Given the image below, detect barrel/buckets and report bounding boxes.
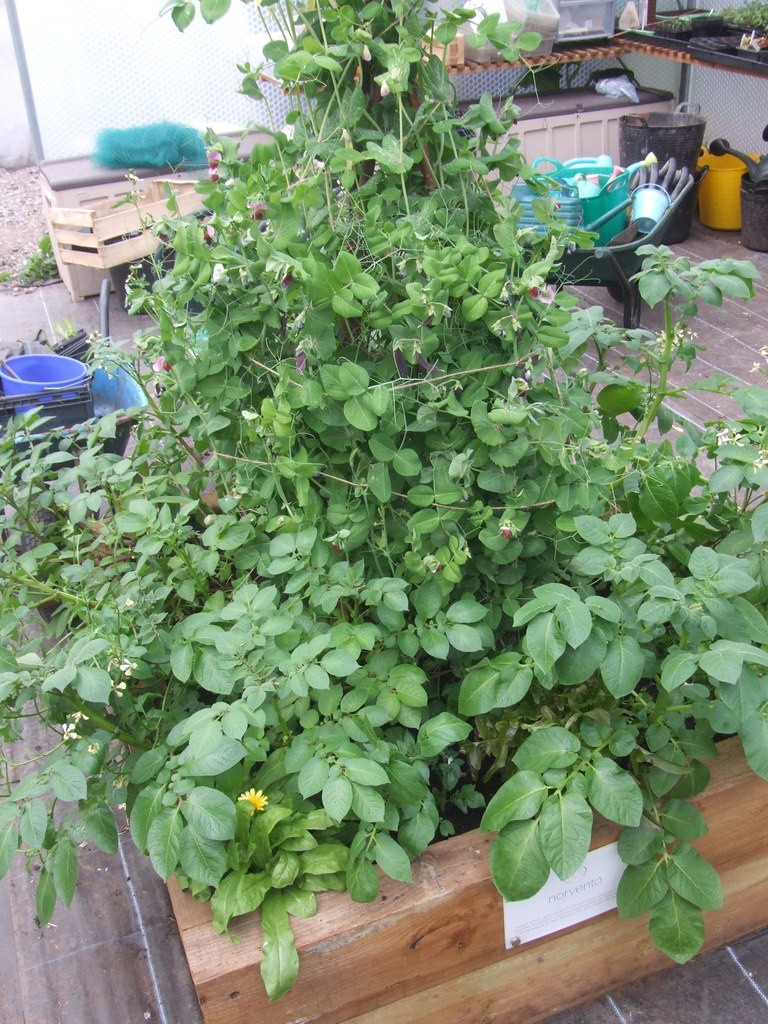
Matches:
[0,354,91,417]
[740,170,768,253]
[696,145,762,231]
[631,165,710,245]
[630,184,671,234]
[531,157,632,247]
[617,101,707,182]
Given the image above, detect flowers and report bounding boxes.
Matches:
[1,0,768,1002]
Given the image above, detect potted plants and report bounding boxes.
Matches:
[651,19,693,42]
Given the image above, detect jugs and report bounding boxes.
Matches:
[710,138,768,182]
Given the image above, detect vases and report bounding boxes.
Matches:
[162,728,766,1024]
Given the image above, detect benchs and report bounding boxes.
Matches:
[37,128,279,303]
[454,82,675,201]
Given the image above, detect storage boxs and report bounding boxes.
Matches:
[47,177,209,268]
[420,0,561,67]
